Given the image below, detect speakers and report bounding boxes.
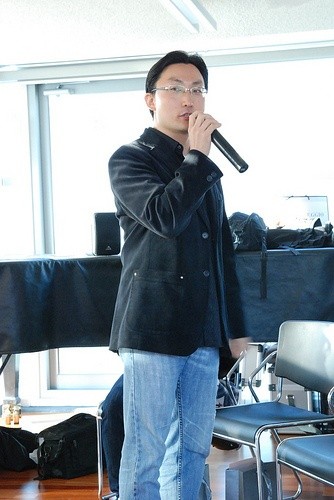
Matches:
[93,212,121,256]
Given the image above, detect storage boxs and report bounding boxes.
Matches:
[225,460,278,500]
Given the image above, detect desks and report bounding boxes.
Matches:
[0,231,334,373]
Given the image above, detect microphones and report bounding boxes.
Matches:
[211,130,248,172]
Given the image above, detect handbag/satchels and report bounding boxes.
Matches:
[0,425,40,471]
[29,412,106,481]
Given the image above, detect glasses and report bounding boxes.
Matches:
[151,84,209,100]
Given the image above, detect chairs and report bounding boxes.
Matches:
[96,320,334,500]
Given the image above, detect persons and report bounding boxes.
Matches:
[108,51,251,500]
